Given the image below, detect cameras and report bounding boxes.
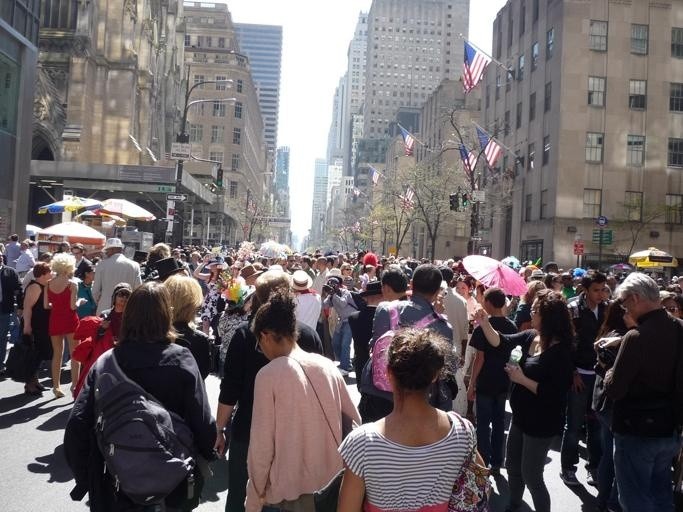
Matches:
[324,285,335,292]
[592,341,618,380]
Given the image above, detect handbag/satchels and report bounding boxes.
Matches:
[6,334,39,382]
[94,374,195,505]
[448,457,492,512]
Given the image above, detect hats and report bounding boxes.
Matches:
[208,257,224,266]
[222,276,256,311]
[289,270,313,291]
[527,269,545,282]
[359,281,382,297]
[101,238,125,253]
[241,265,264,279]
[156,256,184,280]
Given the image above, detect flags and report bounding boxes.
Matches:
[350,187,362,197]
[399,128,415,157]
[462,44,491,93]
[400,187,414,209]
[368,167,381,185]
[460,145,478,175]
[484,140,501,167]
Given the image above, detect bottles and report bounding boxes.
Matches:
[508,345,522,364]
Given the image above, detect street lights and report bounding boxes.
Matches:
[170,79,237,250]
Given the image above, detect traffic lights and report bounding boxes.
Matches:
[216,169,222,187]
[461,192,467,206]
[448,194,458,211]
[470,214,476,227]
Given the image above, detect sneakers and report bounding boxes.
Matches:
[560,471,579,486]
[587,468,600,485]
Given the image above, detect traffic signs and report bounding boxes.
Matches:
[165,193,188,202]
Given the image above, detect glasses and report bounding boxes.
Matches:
[254,332,268,354]
[618,294,639,311]
[666,306,680,313]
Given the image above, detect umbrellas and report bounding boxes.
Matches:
[38,222,106,244]
[103,198,155,222]
[38,196,102,222]
[76,210,129,228]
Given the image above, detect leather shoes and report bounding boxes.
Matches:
[25,385,51,394]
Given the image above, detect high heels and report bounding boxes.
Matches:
[53,388,64,399]
[70,384,77,397]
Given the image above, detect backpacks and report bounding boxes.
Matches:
[373,306,438,393]
